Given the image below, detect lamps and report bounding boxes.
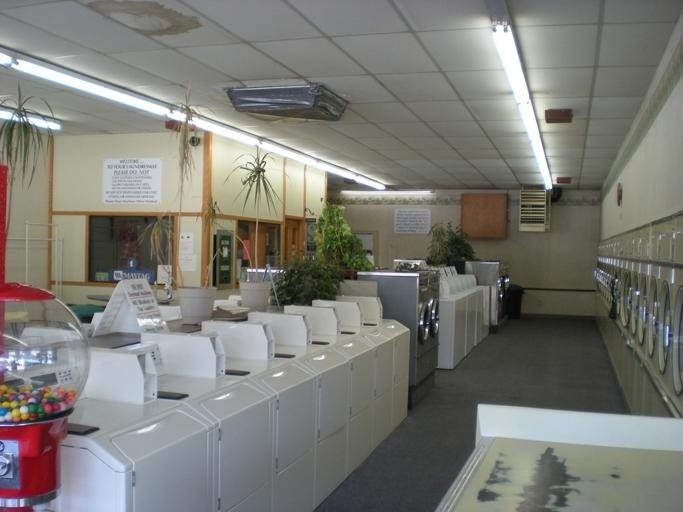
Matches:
[353,176,386,191]
[0,108,62,132]
[530,140,553,191]
[491,23,530,103]
[9,53,171,119]
[0,50,15,68]
[315,161,356,182]
[515,101,541,141]
[169,107,260,147]
[256,138,317,167]
